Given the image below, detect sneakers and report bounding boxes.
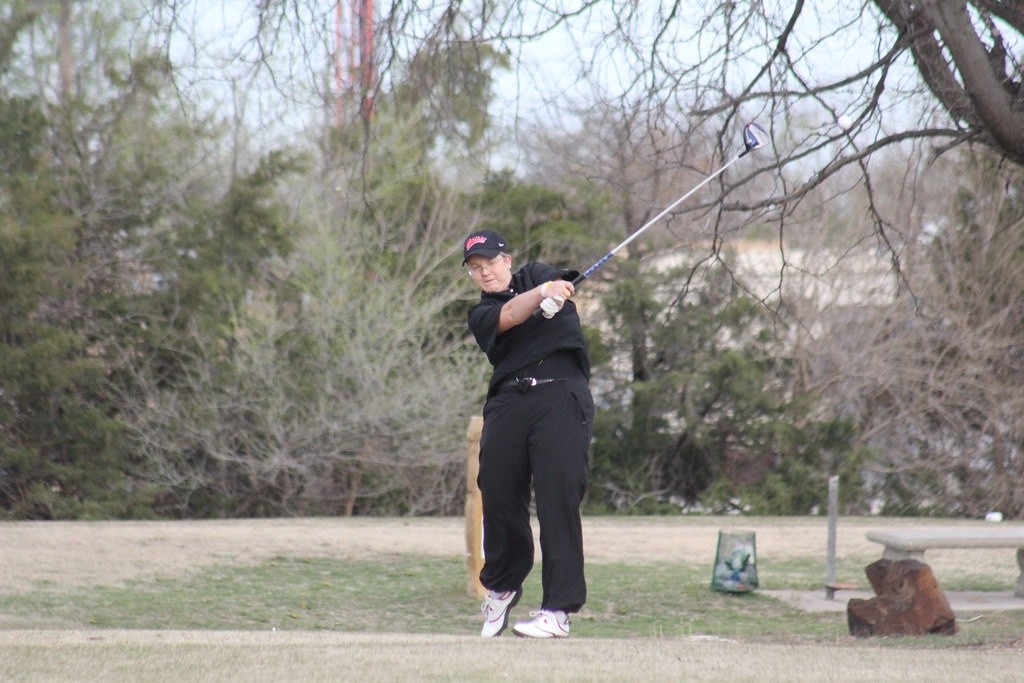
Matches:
[481,586,523,638]
[512,610,570,639]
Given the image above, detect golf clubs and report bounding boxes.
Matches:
[531,122,770,320]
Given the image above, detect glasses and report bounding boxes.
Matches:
[468,257,502,277]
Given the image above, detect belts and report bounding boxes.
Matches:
[503,377,568,387]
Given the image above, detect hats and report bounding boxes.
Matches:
[462,229,507,267]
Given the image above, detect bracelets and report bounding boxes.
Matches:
[541,281,552,298]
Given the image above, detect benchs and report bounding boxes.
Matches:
[864,525,1024,599]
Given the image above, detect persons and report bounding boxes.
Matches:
[461,229,594,638]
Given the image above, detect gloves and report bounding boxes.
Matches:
[540,296,564,319]
